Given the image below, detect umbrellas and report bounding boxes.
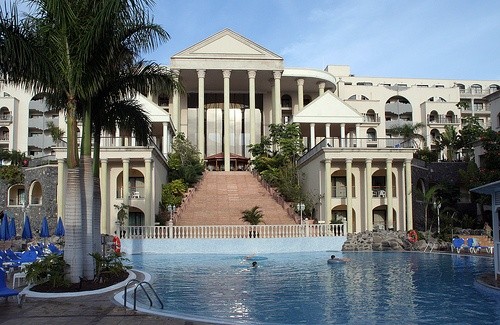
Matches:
[0,211,16,250]
[21,214,33,249]
[39,214,51,252]
[54,217,65,250]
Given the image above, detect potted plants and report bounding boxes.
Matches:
[238,205,267,237]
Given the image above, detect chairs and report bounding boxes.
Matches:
[450,238,494,255]
[0,242,64,307]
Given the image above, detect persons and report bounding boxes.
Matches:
[330,255,351,262]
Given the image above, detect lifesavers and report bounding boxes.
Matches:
[408,230,417,242]
[113,236,120,254]
